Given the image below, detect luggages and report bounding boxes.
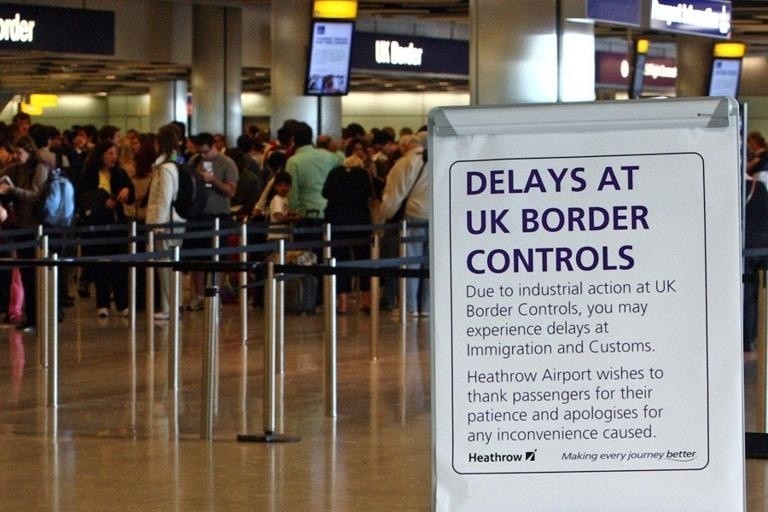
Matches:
[283,274,318,316]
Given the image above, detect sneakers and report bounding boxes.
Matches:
[97,306,130,317]
[58,287,91,322]
[337,303,370,316]
[392,308,431,317]
[0,315,37,331]
[152,298,205,320]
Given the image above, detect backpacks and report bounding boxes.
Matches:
[165,160,207,221]
[42,163,76,229]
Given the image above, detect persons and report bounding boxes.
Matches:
[746,168,767,193]
[0,112,432,333]
[743,148,767,353]
[746,129,768,173]
